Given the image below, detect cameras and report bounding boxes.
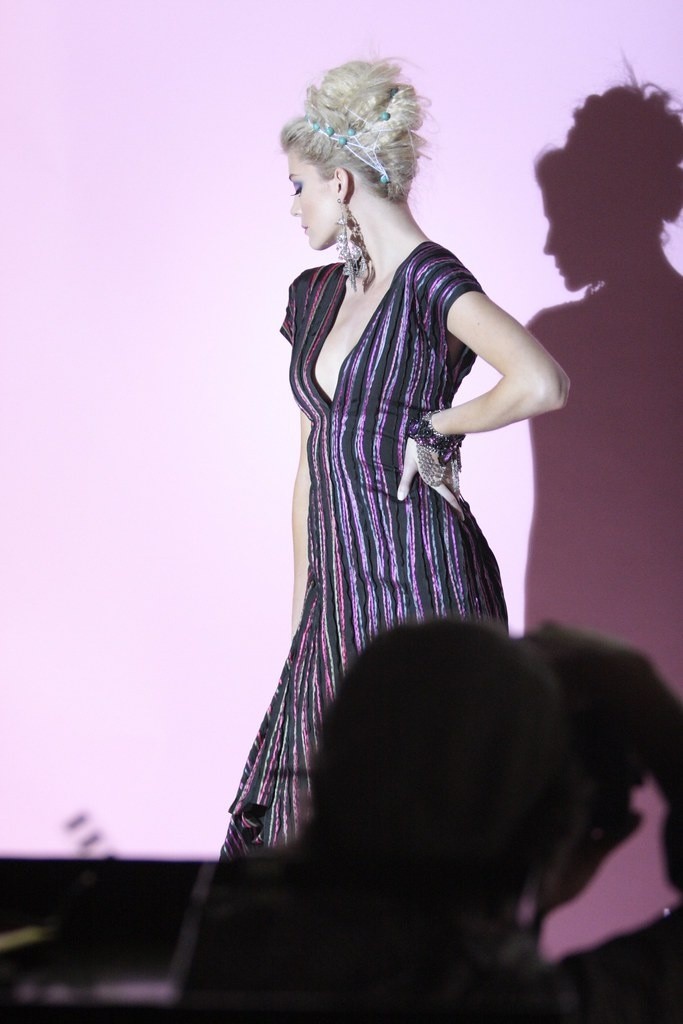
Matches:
[544,664,642,879]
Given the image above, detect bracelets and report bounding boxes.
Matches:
[410,407,466,494]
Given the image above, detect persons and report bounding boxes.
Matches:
[203,55,574,896]
[176,615,683,1024]
